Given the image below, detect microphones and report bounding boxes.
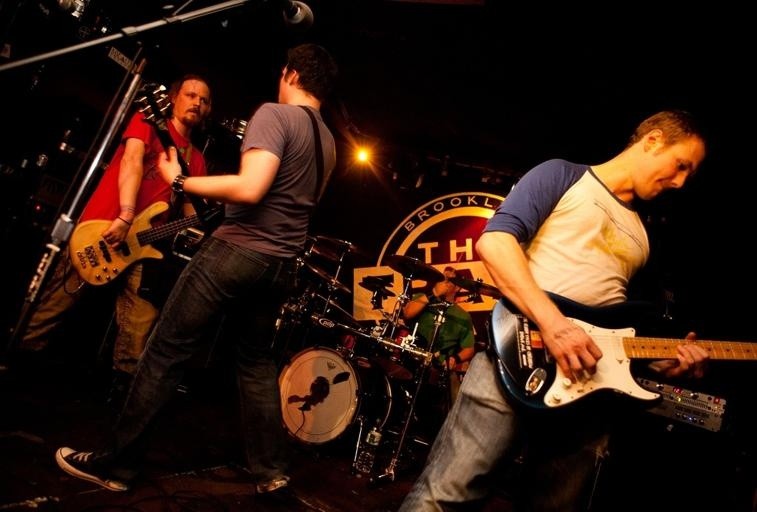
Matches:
[277,0,314,31]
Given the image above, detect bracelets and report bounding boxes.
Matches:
[116,216,133,227]
[450,354,461,365]
[423,289,438,304]
[171,175,188,193]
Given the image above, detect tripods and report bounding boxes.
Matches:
[351,293,475,496]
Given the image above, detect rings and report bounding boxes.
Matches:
[107,237,113,243]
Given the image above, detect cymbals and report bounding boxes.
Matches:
[304,261,351,294]
[377,307,410,329]
[384,254,444,282]
[309,243,339,263]
[308,291,359,327]
[448,277,502,299]
[317,234,374,261]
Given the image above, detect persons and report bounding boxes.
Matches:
[51,41,338,499]
[391,108,712,509]
[398,265,476,387]
[5,75,213,436]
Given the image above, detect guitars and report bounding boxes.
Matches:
[489,290,755,408]
[134,82,224,255]
[70,202,221,286]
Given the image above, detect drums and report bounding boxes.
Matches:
[278,344,393,446]
[355,319,428,380]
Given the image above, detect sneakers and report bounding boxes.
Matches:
[256,475,291,493]
[56,447,129,492]
